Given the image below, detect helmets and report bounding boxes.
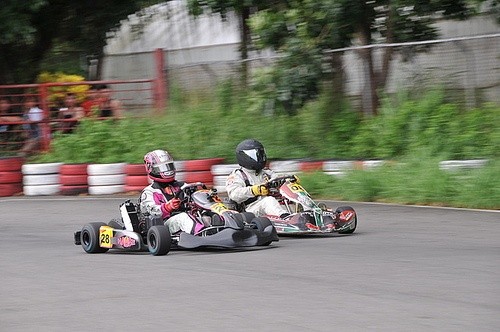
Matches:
[144,150,176,182]
[235,139,267,170]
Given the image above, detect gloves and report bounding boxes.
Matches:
[165,197,180,213]
[252,183,268,196]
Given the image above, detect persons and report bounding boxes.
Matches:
[141,148,205,236]
[224,137,301,218]
[0,85,122,154]
